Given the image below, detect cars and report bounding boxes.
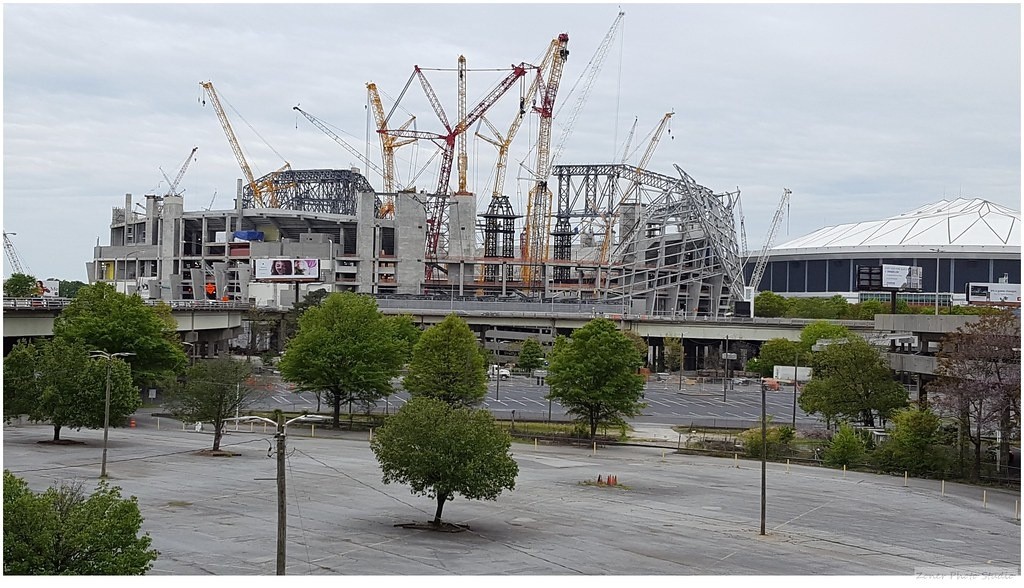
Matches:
[732,377,751,385]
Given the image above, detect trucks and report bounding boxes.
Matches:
[488,365,510,380]
[761,378,783,390]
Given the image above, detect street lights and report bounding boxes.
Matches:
[124,248,146,296]
[491,339,511,400]
[182,240,206,304]
[223,415,335,575]
[87,349,138,477]
[182,341,195,365]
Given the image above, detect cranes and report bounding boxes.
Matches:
[201,7,678,294]
